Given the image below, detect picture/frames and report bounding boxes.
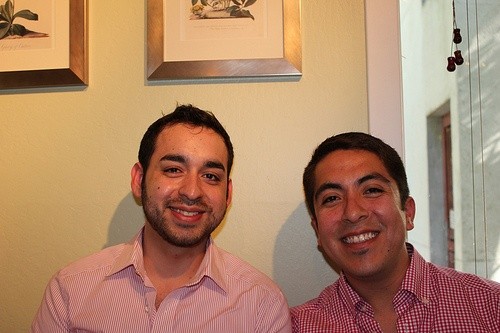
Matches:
[146,0,303,86]
[0,0,89,94]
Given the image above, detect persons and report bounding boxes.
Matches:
[32,104,291,333]
[290,132,500,333]
